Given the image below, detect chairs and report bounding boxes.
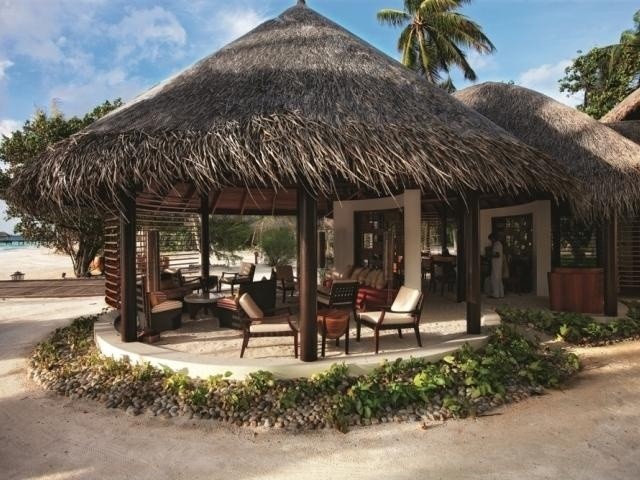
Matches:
[151,263,425,358]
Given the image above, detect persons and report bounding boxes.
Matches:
[254,250,259,265]
[482,232,511,301]
